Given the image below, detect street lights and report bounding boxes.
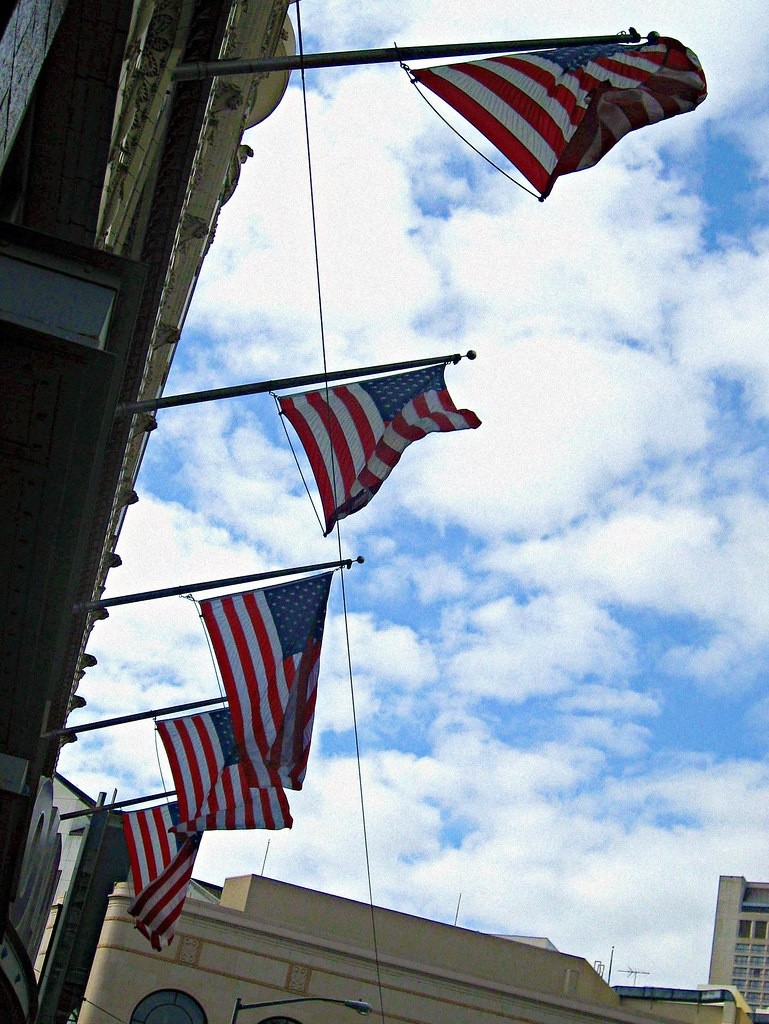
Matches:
[229,997,373,1024]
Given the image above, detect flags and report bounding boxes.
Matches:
[276,366,482,537]
[155,708,294,835]
[410,37,708,201]
[198,572,333,791]
[120,802,203,953]
